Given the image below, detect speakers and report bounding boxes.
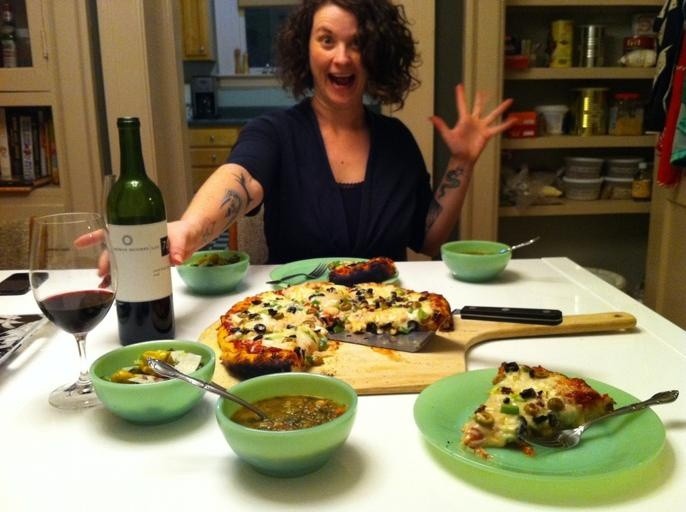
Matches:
[191,75,218,121]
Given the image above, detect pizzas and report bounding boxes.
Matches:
[324,253,398,285]
[216,279,455,371]
[459,358,615,451]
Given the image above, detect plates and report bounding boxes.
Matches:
[413,365,667,482]
[271,257,399,290]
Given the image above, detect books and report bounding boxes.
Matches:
[0,106,59,186]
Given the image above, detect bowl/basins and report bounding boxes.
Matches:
[87,339,216,425]
[177,250,250,293]
[215,371,358,477]
[441,240,511,281]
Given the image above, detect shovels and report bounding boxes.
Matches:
[325,305,563,353]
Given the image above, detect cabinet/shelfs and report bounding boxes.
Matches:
[189,129,240,193]
[0,0,104,271]
[461,1,665,307]
[180,2,217,62]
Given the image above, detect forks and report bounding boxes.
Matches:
[518,389,679,451]
[264,262,329,285]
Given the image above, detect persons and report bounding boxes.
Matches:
[74,0,519,288]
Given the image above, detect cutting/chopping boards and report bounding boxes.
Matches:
[197,312,638,396]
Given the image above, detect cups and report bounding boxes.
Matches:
[29,212,118,411]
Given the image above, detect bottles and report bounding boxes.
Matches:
[105,118,174,346]
[632,161,654,203]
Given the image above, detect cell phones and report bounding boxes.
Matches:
[0,273,48,296]
